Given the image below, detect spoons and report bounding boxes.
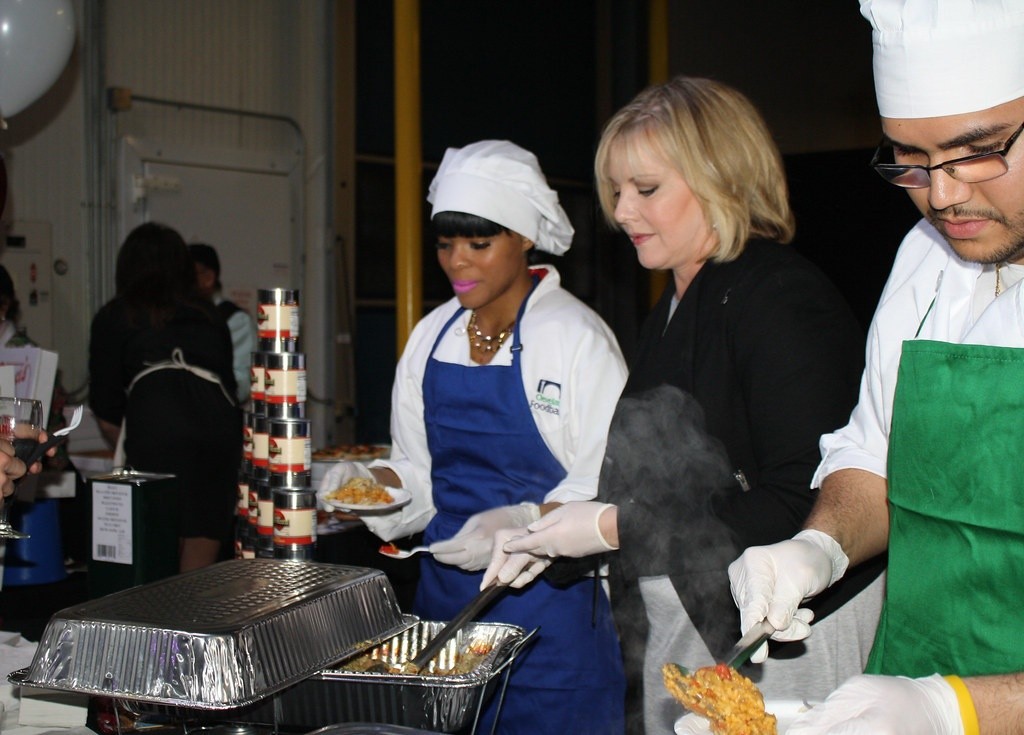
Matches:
[381,547,430,559]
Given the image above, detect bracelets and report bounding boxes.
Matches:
[943,674,979,735]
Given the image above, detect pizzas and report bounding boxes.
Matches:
[312,444,388,460]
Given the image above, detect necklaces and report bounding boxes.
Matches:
[468,312,516,353]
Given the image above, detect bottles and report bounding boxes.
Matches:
[235,288,317,564]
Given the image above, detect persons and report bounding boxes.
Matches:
[314,140,629,735]
[727,0,1024,735]
[480,78,889,735]
[0,264,87,564]
[188,241,256,406]
[86,220,243,575]
[0,422,56,508]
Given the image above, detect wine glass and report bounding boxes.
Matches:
[0,397,43,538]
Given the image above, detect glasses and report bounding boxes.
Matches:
[871,121,1024,189]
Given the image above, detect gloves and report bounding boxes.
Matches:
[728,530,850,663]
[674,672,963,735]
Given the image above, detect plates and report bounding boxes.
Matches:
[313,457,391,461]
[674,700,824,735]
[322,485,412,510]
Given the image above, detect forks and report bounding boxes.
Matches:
[53,404,83,436]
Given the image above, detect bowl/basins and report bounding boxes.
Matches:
[68,456,122,472]
[117,697,218,723]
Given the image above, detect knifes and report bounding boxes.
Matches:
[713,613,774,669]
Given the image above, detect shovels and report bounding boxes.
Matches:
[365,561,536,674]
[663,598,815,719]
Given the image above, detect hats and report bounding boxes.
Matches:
[858,0,1024,118]
[427,140,575,256]
